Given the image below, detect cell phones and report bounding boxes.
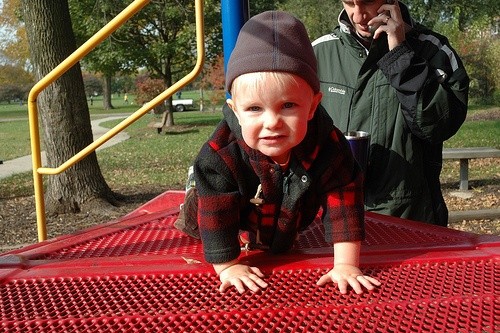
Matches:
[368,0,398,34]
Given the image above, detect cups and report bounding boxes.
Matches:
[341,130,371,182]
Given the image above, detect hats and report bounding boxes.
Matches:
[226,10,321,95]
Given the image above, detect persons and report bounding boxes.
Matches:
[174,11,382,295]
[176,90,182,99]
[125,93,128,104]
[310,0,469,227]
[90,96,93,105]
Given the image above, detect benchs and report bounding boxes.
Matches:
[442,146,500,191]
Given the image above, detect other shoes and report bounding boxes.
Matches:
[186,166,197,193]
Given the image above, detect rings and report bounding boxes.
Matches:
[385,16,391,24]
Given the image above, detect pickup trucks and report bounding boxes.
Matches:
[142,98,194,114]
